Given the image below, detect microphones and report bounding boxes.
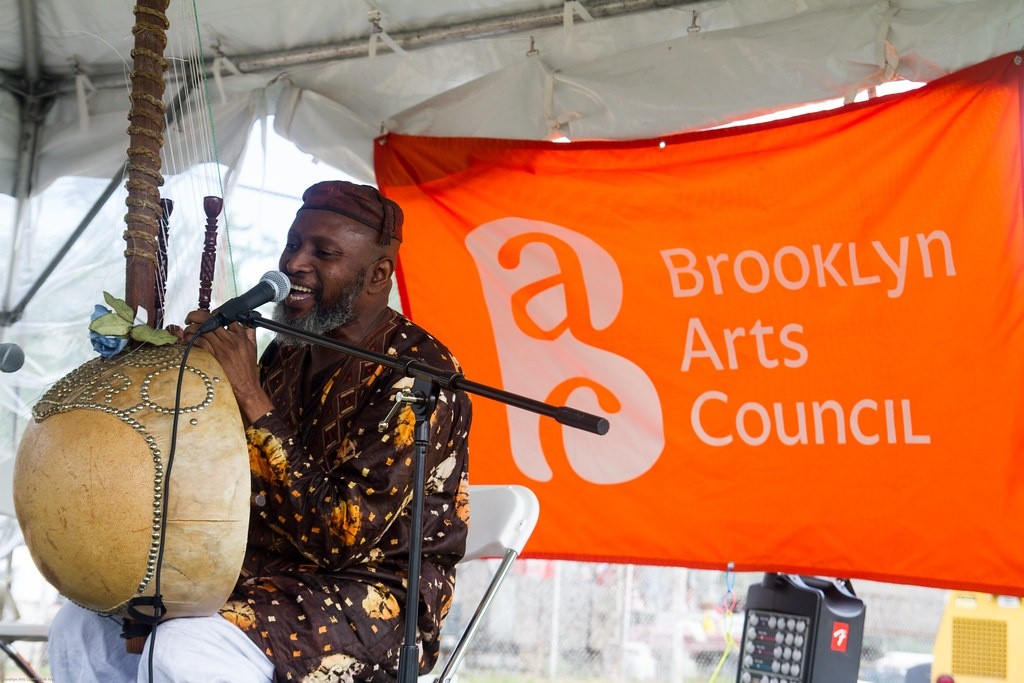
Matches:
[0,343,25,373]
[195,271,291,335]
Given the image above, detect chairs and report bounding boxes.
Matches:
[418,483,542,683]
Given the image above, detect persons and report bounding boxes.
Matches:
[49,181,473,683]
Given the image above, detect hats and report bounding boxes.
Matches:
[297,181,404,246]
